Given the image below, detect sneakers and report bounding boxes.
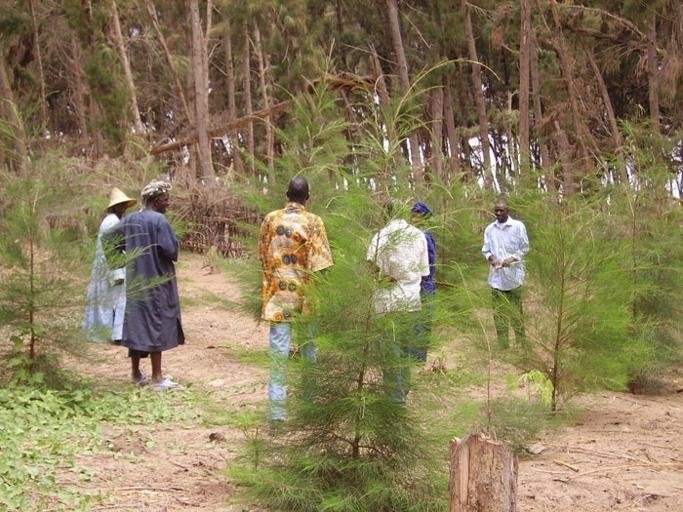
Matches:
[136,374,179,391]
[270,420,288,437]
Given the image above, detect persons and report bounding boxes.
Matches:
[367,203,431,406]
[410,202,436,365]
[481,200,530,350]
[258,176,335,432]
[102,180,178,390]
[86,188,136,345]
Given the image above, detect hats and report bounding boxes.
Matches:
[141,181,172,199]
[412,202,433,217]
[104,187,137,213]
